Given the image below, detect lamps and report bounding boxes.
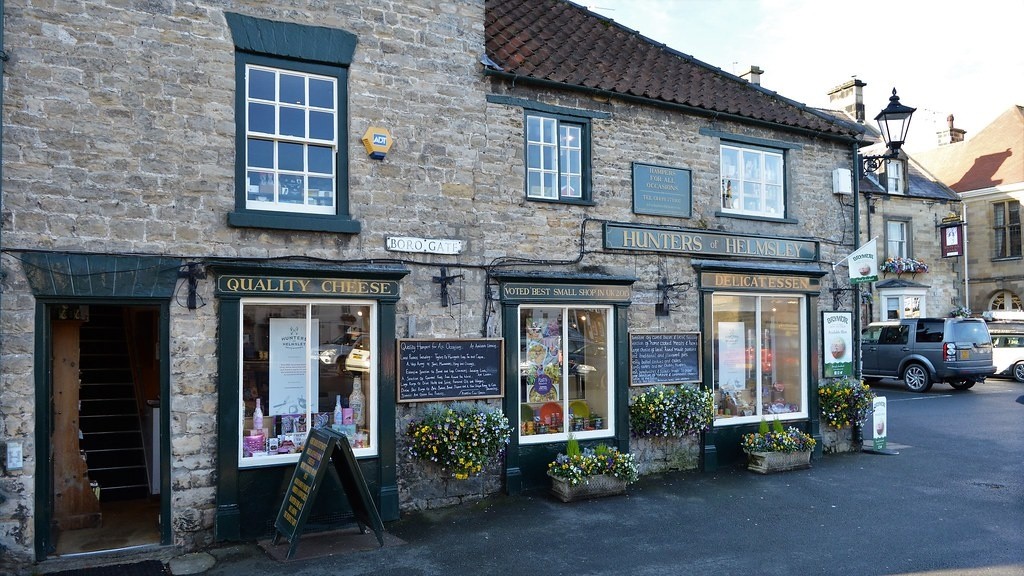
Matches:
[852,87,917,182]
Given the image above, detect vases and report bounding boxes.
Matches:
[253,398,263,429]
[725,408,730,415]
[348,376,366,426]
[743,449,811,474]
[546,471,627,502]
[887,266,922,274]
[719,409,724,415]
[334,395,343,424]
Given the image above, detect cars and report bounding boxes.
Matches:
[987,335,1024,381]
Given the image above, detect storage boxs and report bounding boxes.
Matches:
[343,408,354,424]
[316,413,330,427]
[332,424,356,444]
[244,428,269,451]
[273,414,300,436]
[243,435,263,456]
[287,432,306,447]
[268,438,278,451]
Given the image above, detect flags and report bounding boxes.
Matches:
[849,240,878,283]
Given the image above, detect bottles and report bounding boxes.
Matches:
[718,406,724,415]
[349,376,366,427]
[334,395,342,424]
[714,404,718,416]
[253,398,263,429]
[569,407,574,419]
[551,412,560,425]
[590,408,595,425]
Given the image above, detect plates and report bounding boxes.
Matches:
[773,381,784,392]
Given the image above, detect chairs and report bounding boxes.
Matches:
[999,337,1010,347]
[1018,337,1024,347]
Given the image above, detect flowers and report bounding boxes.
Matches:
[880,256,930,275]
[401,399,517,479]
[950,304,974,318]
[861,291,881,305]
[725,395,731,408]
[546,431,640,486]
[817,375,878,432]
[628,382,718,444]
[719,401,723,409]
[741,413,817,455]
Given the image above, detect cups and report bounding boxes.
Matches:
[725,408,731,415]
[342,408,353,424]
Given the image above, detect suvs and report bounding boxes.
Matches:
[861,316,995,391]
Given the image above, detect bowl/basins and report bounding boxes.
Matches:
[569,400,589,419]
[540,402,563,424]
